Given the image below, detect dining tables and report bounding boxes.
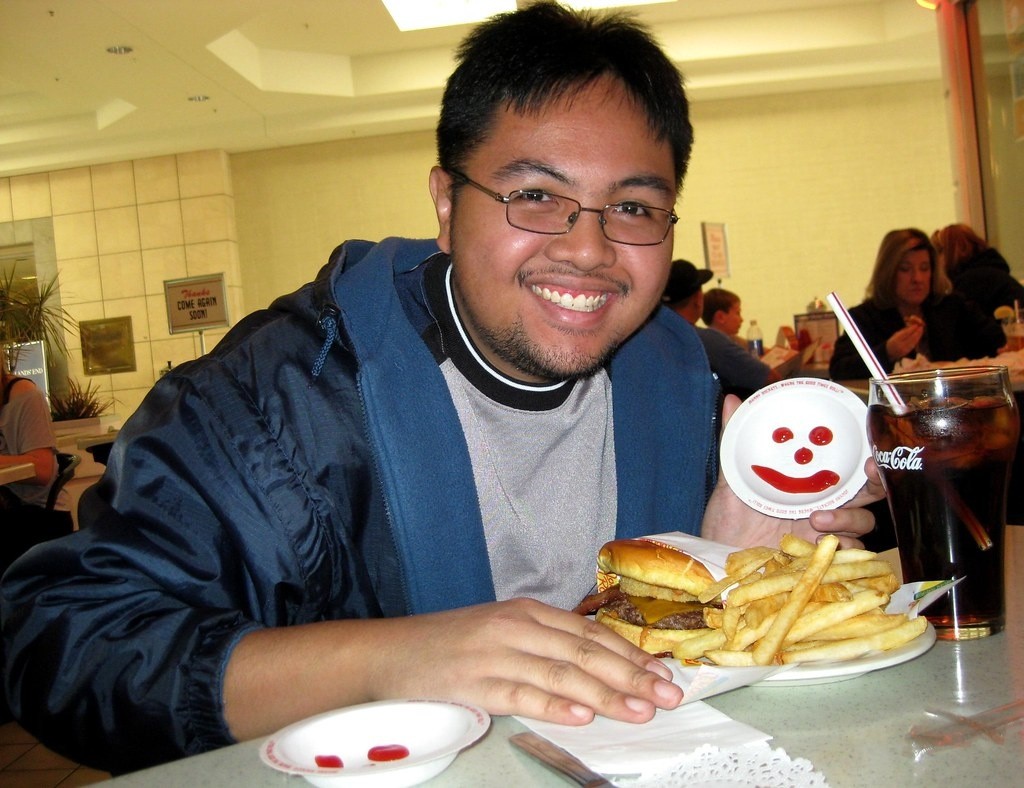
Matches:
[84,614,1024,788]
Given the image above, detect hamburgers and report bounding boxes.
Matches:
[570,539,720,658]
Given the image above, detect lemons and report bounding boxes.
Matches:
[994,306,1014,319]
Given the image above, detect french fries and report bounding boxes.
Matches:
[673,534,927,664]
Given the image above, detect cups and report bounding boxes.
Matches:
[1002,310,1024,349]
[866,365,1019,643]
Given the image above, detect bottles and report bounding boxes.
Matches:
[747,318,763,359]
[820,342,832,364]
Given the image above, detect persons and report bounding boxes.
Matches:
[827,224,1024,380]
[0,352,75,572]
[0,0,885,773]
[661,258,783,403]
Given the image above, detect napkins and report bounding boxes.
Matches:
[509,699,776,777]
[656,575,968,707]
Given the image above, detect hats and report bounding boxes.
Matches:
[660,259,714,305]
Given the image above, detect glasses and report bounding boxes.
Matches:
[445,169,678,246]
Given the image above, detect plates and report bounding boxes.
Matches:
[259,697,490,787]
[746,621,937,687]
[723,377,871,519]
